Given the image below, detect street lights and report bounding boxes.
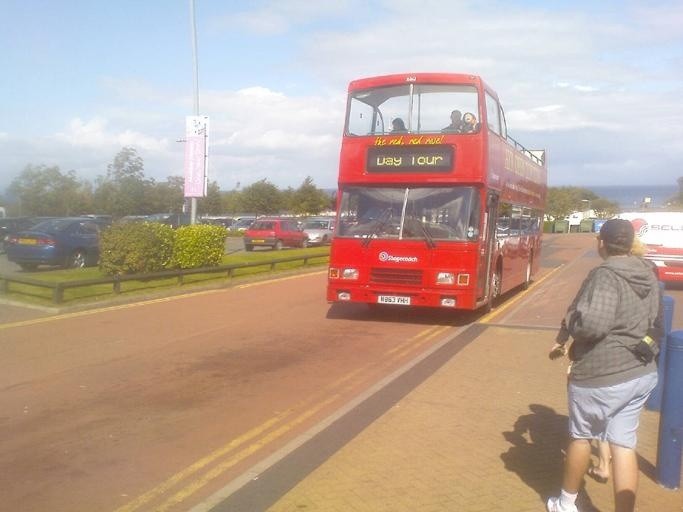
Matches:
[580,199,591,218]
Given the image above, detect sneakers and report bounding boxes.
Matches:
[547,497,579,512]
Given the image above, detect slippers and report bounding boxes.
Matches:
[588,467,610,484]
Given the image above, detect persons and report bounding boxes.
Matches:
[389,117,409,134]
[547,235,646,486]
[457,111,480,133]
[545,220,662,512]
[439,109,463,132]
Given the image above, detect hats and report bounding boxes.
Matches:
[599,218,634,245]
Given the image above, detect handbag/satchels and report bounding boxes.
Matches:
[634,329,661,363]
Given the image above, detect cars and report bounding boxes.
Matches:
[79,213,237,229]
[226,218,257,231]
[298,212,356,246]
[0,217,48,255]
[3,218,110,270]
[243,217,310,251]
[613,212,683,289]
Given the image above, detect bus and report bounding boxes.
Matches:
[326,71,549,312]
